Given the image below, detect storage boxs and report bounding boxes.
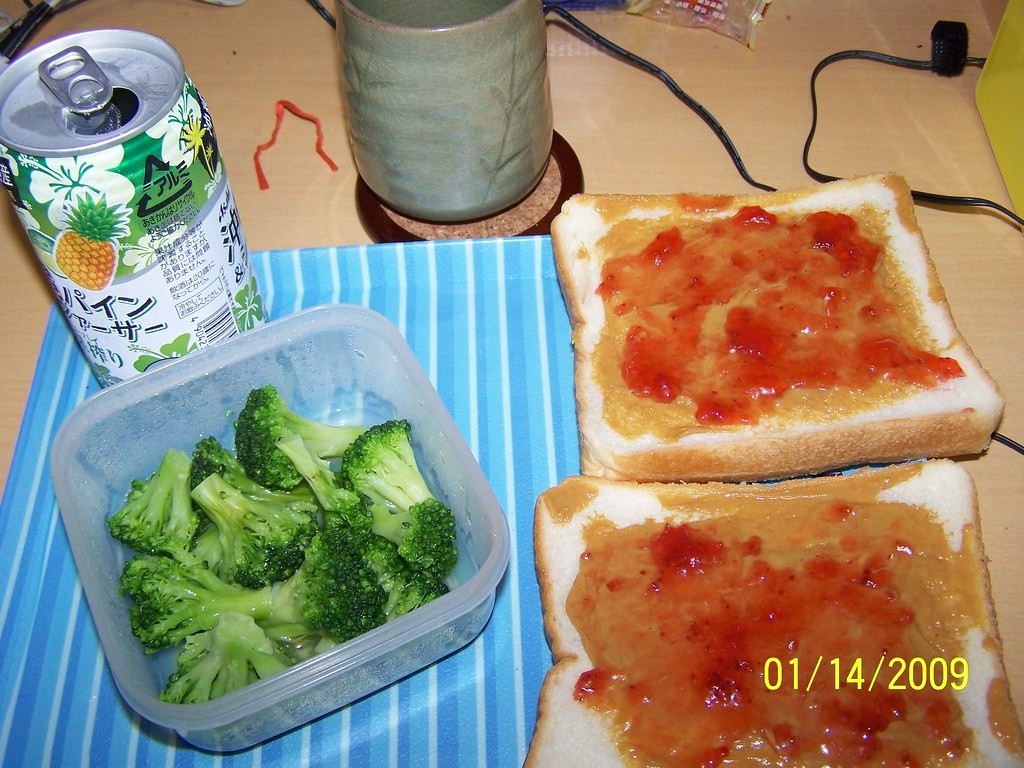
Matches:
[48,304,511,755]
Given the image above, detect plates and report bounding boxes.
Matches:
[0,236,954,768]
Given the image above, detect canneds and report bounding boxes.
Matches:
[0,29,269,393]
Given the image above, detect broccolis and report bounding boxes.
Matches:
[110,386,457,712]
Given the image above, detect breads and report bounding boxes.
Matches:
[522,457,1024,768]
[552,195,1003,483]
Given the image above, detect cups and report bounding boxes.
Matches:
[335,1,555,224]
